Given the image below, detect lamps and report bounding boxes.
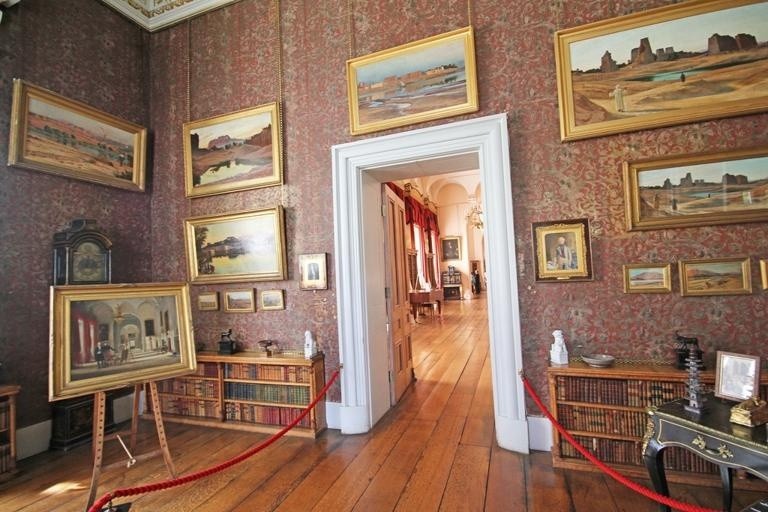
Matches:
[464,194,483,231]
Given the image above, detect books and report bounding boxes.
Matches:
[149,362,311,428]
[556,377,768,475]
[0,399,10,474]
[442,267,462,284]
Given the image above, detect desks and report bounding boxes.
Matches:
[0,384,21,475]
[441,284,463,300]
[641,391,767,512]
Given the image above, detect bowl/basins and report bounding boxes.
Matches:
[581,353,615,368]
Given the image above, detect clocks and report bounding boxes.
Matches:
[48,219,117,451]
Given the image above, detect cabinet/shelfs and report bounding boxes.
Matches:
[142,347,327,438]
[546,361,767,492]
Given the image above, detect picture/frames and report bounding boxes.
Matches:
[440,237,461,261]
[345,25,486,136]
[48,281,197,403]
[714,350,761,403]
[183,101,286,199]
[552,0,768,142]
[198,291,218,311]
[760,259,768,290]
[679,256,752,296]
[532,218,594,282]
[183,205,288,285]
[8,78,146,192]
[622,262,671,294]
[224,288,255,313]
[260,289,285,310]
[299,253,327,291]
[622,147,768,231]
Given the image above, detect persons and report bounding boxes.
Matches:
[94,341,128,368]
[470,272,475,294]
[655,194,659,210]
[475,270,480,294]
[670,196,678,210]
[609,85,626,112]
[556,236,573,269]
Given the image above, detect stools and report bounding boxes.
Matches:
[419,303,437,319]
